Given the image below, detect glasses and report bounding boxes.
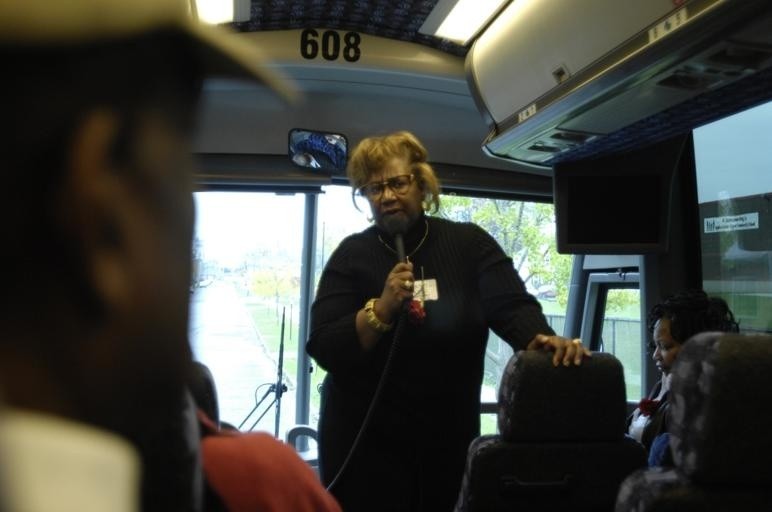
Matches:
[362,174,420,202]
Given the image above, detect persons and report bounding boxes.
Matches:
[617,293,738,441]
[4,0,298,508]
[180,358,342,509]
[303,127,591,511]
[291,130,345,170]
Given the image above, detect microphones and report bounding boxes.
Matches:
[380,207,413,311]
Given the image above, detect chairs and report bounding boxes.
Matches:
[193,336,772,512]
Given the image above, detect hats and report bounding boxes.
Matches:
[0,0,302,108]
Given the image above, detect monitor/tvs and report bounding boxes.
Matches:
[554,163,672,254]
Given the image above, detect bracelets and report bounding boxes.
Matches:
[364,300,396,333]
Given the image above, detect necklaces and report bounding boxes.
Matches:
[376,217,430,261]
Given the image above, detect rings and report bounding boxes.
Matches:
[572,337,582,348]
[402,278,415,290]
